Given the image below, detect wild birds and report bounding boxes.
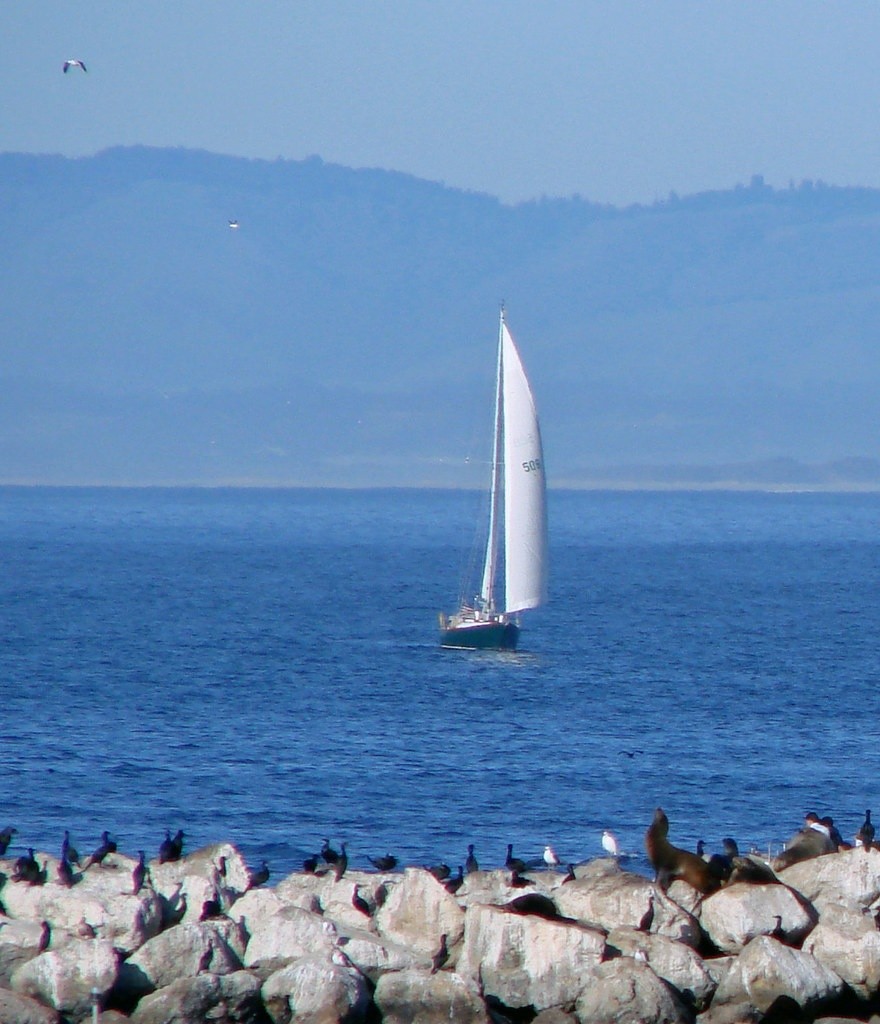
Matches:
[1,809,874,976]
[63,59,87,74]
[229,220,238,228]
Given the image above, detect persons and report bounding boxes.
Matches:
[806,812,843,848]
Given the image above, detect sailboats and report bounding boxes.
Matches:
[435,298,553,654]
